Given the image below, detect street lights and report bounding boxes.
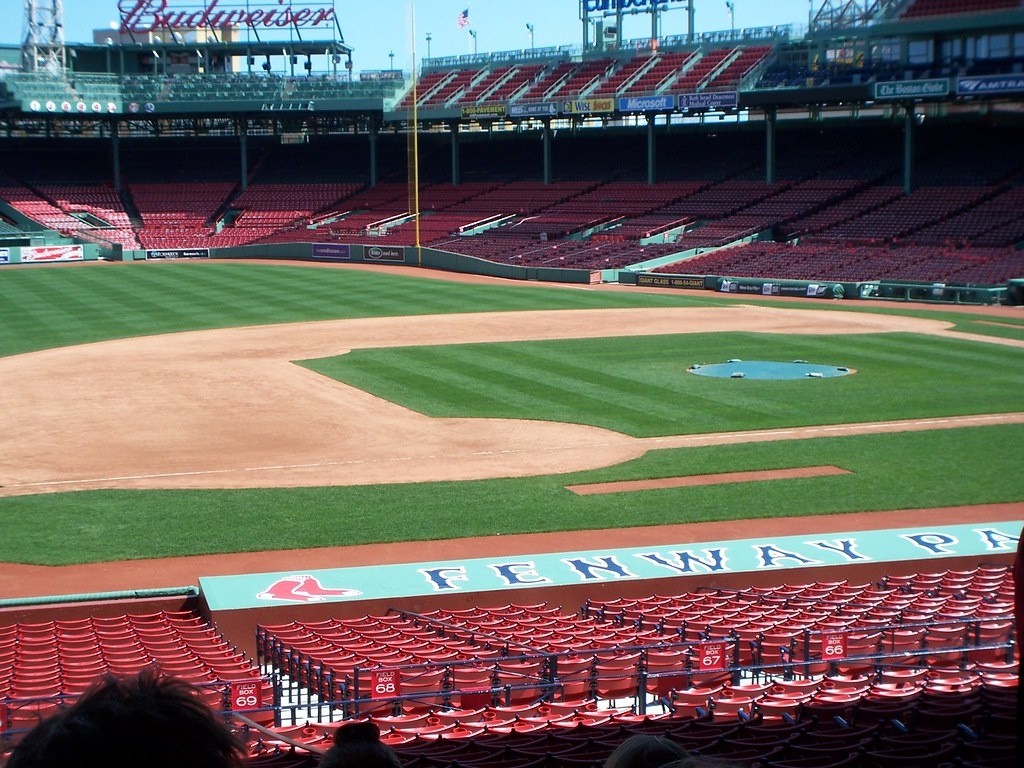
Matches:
[526,23,536,58]
[389,49,394,70]
[469,30,477,54]
[426,32,432,57]
[726,0,735,41]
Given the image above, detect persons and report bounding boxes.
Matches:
[7,668,247,768]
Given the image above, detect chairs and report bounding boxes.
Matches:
[5,75,405,109]
[397,44,776,114]
[0,183,1024,284]
[0,564,1024,768]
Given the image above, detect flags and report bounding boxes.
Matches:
[458,9,468,29]
